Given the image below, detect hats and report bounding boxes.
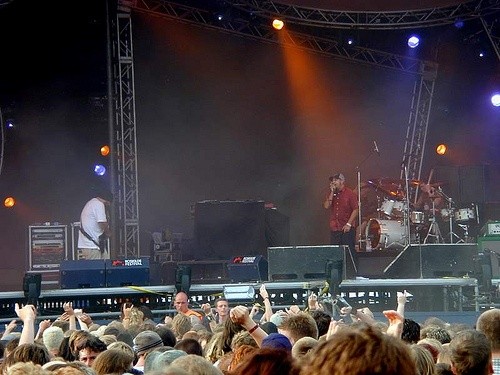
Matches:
[97,189,113,201]
[261,332,292,353]
[133,330,164,357]
[329,173,345,183]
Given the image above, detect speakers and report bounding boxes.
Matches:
[105,258,150,287]
[226,254,269,282]
[58,259,106,288]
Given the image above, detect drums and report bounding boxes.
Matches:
[377,199,394,216]
[453,208,477,224]
[392,201,409,214]
[365,217,408,251]
[408,210,426,223]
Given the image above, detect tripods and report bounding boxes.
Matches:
[423,191,445,244]
[440,191,464,245]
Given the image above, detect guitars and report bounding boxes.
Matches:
[99,220,112,253]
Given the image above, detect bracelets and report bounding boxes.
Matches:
[264,297,269,301]
[249,324,259,334]
[347,223,352,227]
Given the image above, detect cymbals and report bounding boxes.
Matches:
[425,182,446,186]
[381,180,405,193]
[400,179,422,185]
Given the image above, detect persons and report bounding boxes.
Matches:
[0,284,500,375]
[323,172,359,273]
[412,179,443,244]
[77,190,114,260]
